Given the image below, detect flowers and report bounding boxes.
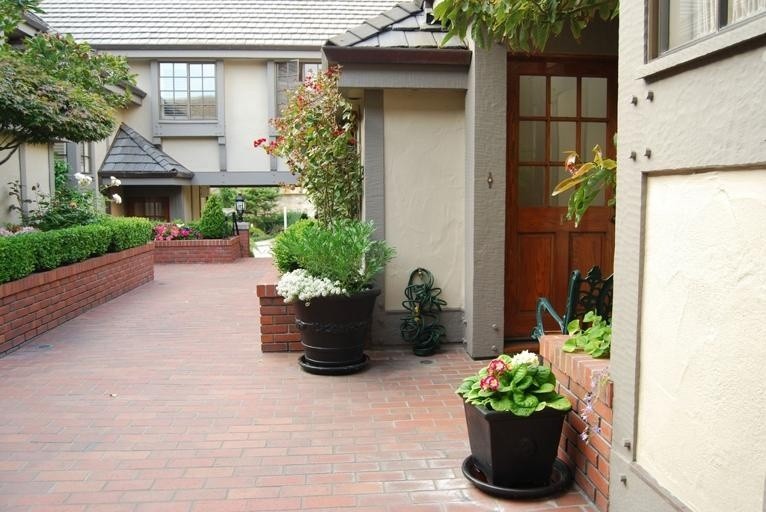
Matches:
[454,348,573,418]
[276,268,346,307]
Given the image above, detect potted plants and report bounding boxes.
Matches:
[276,213,385,377]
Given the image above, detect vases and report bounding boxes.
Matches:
[458,392,574,501]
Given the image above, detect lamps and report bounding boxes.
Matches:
[234,193,246,222]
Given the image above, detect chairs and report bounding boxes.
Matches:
[532,264,612,364]
